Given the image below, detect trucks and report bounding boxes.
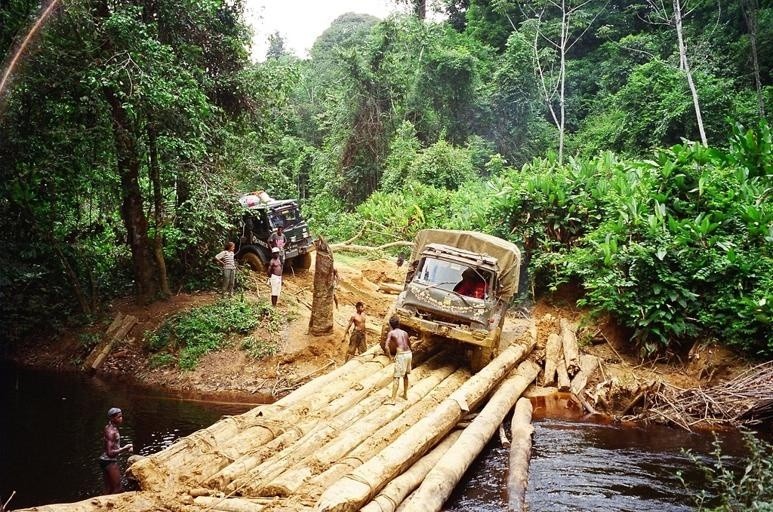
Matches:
[378,226,522,376]
[141,190,314,275]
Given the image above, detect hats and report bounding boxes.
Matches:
[107,408,121,418]
[272,247,280,253]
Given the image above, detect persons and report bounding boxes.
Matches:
[99,407,133,495]
[342,302,367,363]
[267,225,287,271]
[384,316,412,405]
[215,242,236,299]
[267,246,282,306]
